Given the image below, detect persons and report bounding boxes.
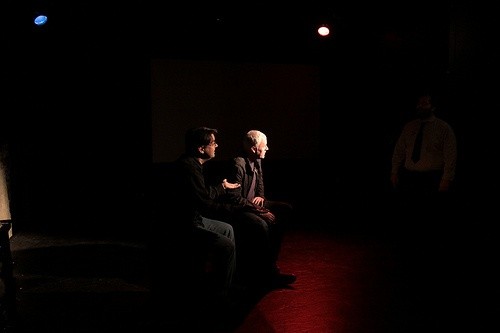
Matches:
[222,129,301,284]
[161,126,249,311]
[390,93,457,267]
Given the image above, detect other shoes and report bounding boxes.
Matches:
[269,268,297,286]
